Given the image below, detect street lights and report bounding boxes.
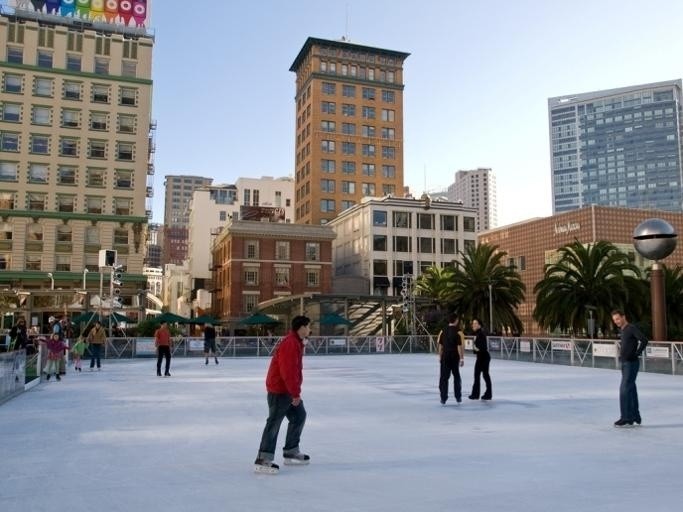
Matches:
[47,272,54,290]
[486,275,495,335]
[583,305,596,340]
[83,268,90,291]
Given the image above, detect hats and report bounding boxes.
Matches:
[49,316,56,323]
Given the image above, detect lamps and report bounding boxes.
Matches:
[400,273,408,313]
[109,264,124,337]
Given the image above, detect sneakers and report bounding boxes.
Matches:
[79,368,81,372]
[469,395,479,399]
[46,374,50,380]
[634,419,641,424]
[614,420,633,424]
[75,367,77,370]
[165,372,171,376]
[254,459,280,470]
[440,399,446,403]
[481,395,492,400]
[157,373,161,375]
[56,374,60,380]
[282,453,310,461]
[456,397,462,402]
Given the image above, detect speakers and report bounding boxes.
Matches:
[98,249,118,267]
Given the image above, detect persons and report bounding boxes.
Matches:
[36,332,70,381]
[611,308,648,427]
[47,315,71,375]
[469,318,494,400]
[436,313,465,404]
[203,323,218,364]
[85,320,106,367]
[71,335,87,370]
[154,320,170,377]
[253,315,310,472]
[7,318,27,380]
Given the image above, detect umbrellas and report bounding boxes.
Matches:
[148,311,190,324]
[104,311,130,322]
[182,314,221,325]
[237,313,281,336]
[311,313,354,328]
